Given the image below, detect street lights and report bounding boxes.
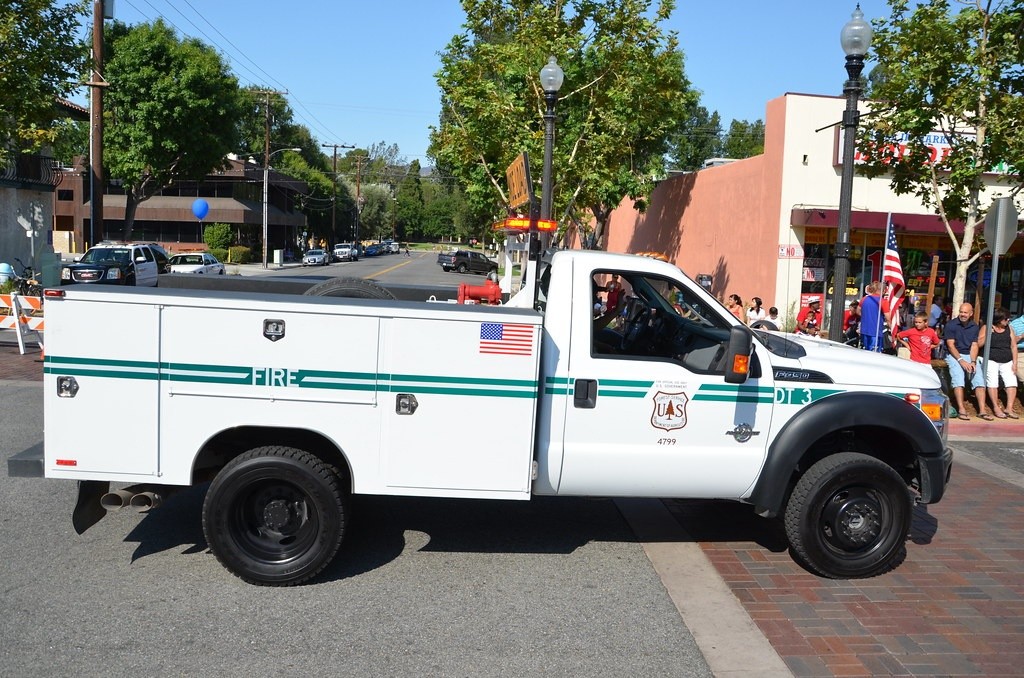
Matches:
[262,148,302,268]
[828,5,874,344]
[538,55,565,294]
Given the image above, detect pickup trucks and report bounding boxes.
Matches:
[9,242,955,587]
[436,251,498,275]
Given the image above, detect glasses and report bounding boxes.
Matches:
[1002,318,1007,321]
[614,277,618,279]
[853,307,857,309]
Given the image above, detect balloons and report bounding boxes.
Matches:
[191,198,209,221]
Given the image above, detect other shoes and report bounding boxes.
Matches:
[994,412,1007,419]
[1005,413,1019,419]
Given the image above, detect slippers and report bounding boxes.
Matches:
[976,414,994,420]
[958,414,970,420]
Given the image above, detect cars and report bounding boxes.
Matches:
[364,245,384,256]
[301,249,329,266]
[168,253,226,276]
[381,241,400,255]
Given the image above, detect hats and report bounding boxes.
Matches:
[849,300,858,305]
[808,296,821,303]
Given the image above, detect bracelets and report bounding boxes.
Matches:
[971,360,976,365]
[957,357,963,362]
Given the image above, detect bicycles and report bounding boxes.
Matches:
[13,257,42,304]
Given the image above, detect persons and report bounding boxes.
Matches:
[943,302,995,422]
[592,273,663,357]
[726,294,745,325]
[605,274,622,320]
[746,296,783,332]
[793,296,823,340]
[403,242,413,257]
[977,307,1020,420]
[843,279,1024,421]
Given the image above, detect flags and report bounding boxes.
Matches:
[877,213,906,350]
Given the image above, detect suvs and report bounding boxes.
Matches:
[332,242,357,262]
[58,242,170,287]
